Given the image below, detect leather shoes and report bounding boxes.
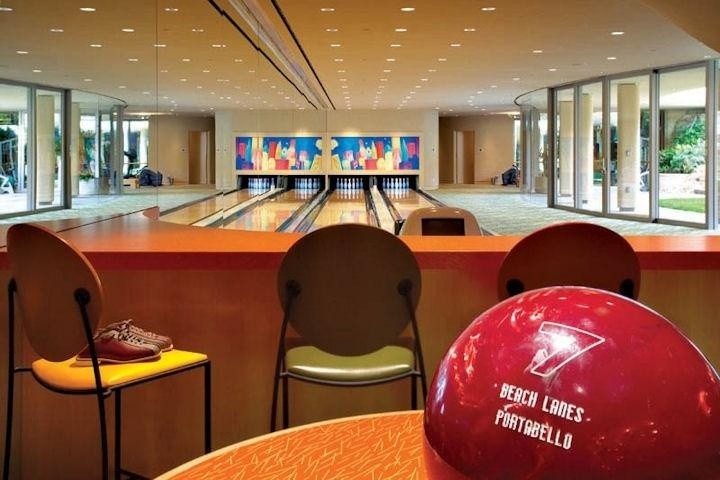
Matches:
[75,330,162,366]
[98,320,173,352]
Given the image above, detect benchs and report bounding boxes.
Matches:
[124,178,140,189]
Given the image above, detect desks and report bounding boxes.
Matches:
[156,409,427,478]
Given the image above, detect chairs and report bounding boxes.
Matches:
[271,223,428,428]
[497,224,639,299]
[395,208,482,236]
[2,224,212,479]
